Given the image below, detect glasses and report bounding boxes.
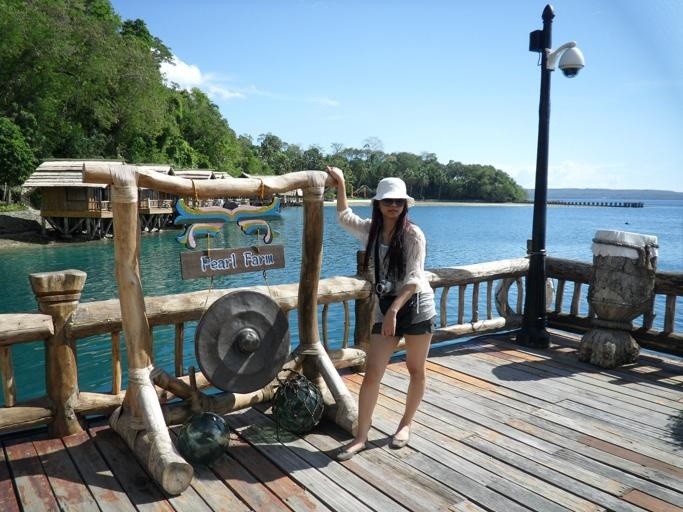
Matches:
[382,198,404,206]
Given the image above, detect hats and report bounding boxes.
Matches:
[371,177,415,208]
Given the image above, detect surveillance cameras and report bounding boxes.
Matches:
[558,46,585,78]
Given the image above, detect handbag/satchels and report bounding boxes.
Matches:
[379,293,420,319]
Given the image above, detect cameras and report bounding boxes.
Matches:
[376,280,392,295]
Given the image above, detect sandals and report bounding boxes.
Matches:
[336,439,368,459]
[390,425,410,448]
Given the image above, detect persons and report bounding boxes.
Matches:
[325,166,437,461]
